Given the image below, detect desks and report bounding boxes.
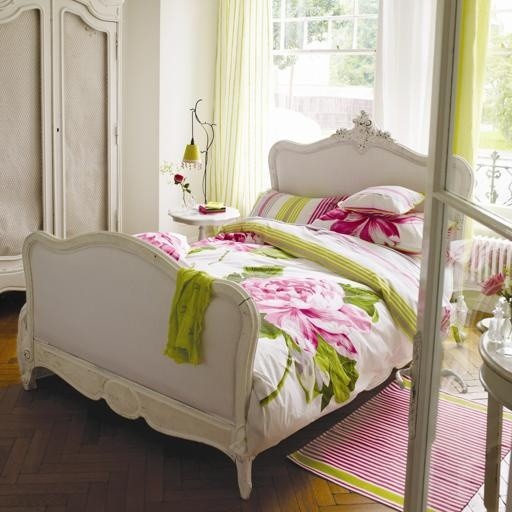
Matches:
[168,203,241,242]
[476,330,512,512]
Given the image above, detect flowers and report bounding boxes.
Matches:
[174,173,191,208]
[478,263,512,323]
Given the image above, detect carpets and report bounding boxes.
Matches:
[285,375,510,511]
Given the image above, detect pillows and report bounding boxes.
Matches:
[249,182,456,255]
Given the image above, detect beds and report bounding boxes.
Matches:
[16,109,474,499]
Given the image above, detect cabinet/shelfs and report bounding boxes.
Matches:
[0,2,125,292]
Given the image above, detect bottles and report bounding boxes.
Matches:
[486,303,506,344]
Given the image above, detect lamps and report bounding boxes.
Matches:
[182,98,227,214]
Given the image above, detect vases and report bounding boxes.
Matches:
[180,194,194,213]
